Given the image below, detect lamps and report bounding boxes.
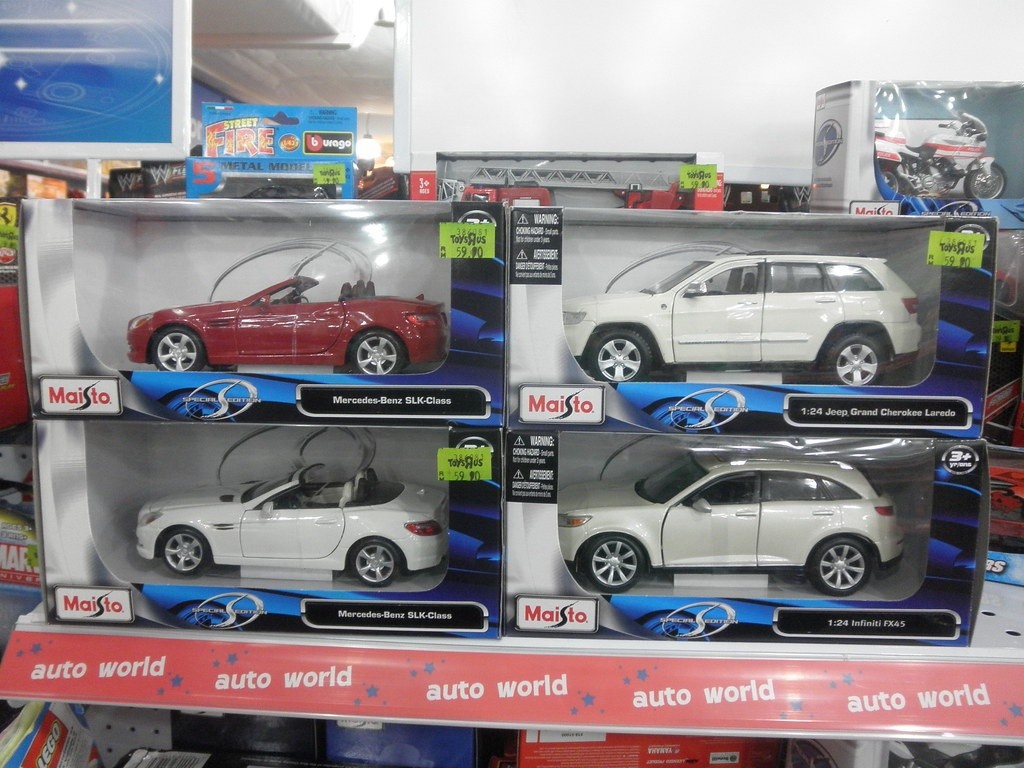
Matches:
[357,114,382,160]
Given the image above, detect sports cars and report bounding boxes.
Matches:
[123,277,457,380]
[134,461,451,588]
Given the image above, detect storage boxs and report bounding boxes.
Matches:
[187,102,357,199]
[30,420,504,639]
[505,206,997,440]
[813,81,1024,213]
[170,709,503,767]
[18,198,509,426]
[984,231,1023,587]
[109,160,187,198]
[517,730,785,768]
[505,432,993,647]
[786,738,1024,768]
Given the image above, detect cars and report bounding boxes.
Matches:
[556,449,908,599]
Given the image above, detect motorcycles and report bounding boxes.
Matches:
[874,113,1009,206]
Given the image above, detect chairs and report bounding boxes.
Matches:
[338,280,375,301]
[339,468,378,507]
[743,272,755,293]
[799,276,869,291]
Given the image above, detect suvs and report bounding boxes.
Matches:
[572,250,930,387]
